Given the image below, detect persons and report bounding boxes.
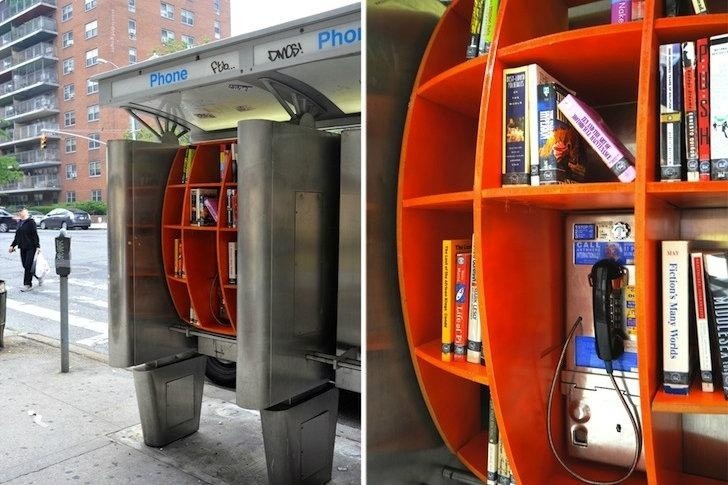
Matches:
[9,206,44,292]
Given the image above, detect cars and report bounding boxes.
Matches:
[28,210,48,226]
[0,206,20,232]
[39,207,92,230]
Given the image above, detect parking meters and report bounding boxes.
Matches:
[53,234,73,374]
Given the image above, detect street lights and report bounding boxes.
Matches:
[96,58,138,140]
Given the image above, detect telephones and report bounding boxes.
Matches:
[591,259,623,361]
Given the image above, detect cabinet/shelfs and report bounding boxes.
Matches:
[393,0,727,484]
[160,138,239,341]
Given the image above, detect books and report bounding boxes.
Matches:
[174,143,237,327]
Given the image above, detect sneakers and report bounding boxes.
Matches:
[20,285,33,293]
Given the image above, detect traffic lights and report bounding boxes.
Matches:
[41,135,48,150]
